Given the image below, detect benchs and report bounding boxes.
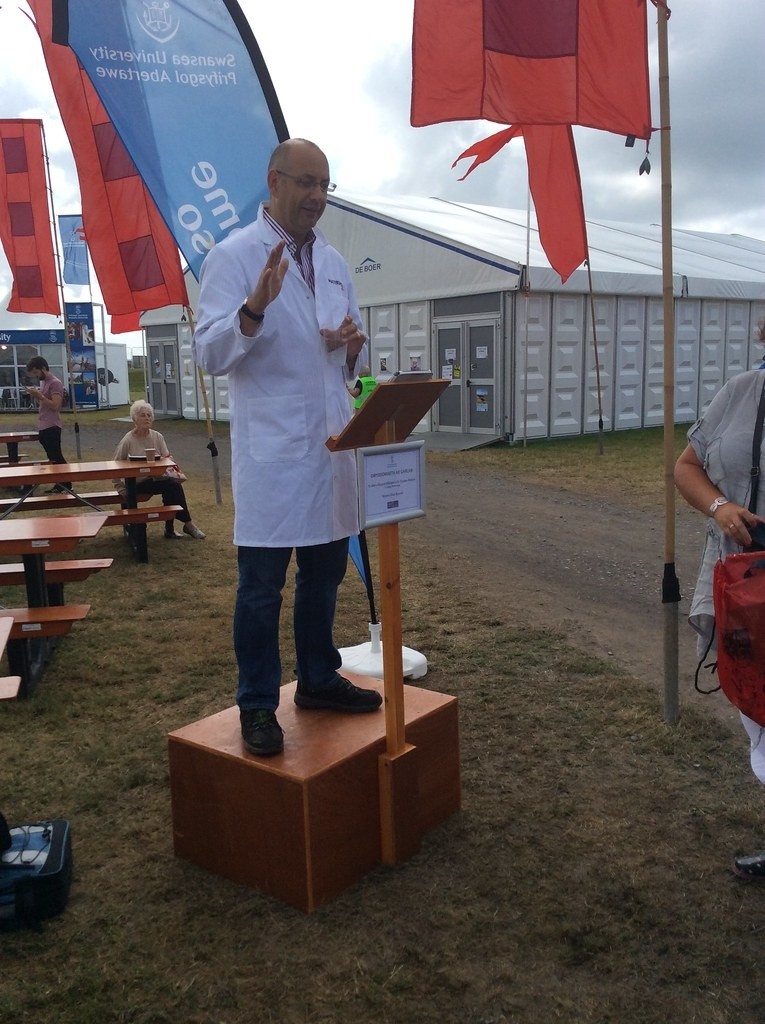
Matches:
[0,675,22,700]
[0,558,114,602]
[0,459,52,495]
[0,488,153,539]
[21,504,184,562]
[0,454,28,462]
[0,604,91,699]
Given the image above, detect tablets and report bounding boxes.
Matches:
[128,453,161,458]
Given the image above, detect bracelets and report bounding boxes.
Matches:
[347,387,351,391]
[706,496,731,522]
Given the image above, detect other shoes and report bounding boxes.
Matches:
[164,529,183,539]
[182,526,204,538]
[732,853,764,881]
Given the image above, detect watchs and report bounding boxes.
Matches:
[239,295,266,322]
[38,395,44,401]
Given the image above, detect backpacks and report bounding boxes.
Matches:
[0,817,72,928]
[61,387,68,407]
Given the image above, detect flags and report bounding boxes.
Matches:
[109,311,149,335]
[448,113,588,287]
[51,2,293,285]
[57,215,91,285]
[27,1,193,312]
[409,0,653,142]
[1,118,61,317]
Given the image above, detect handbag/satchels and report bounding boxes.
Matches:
[161,462,185,485]
[710,549,765,727]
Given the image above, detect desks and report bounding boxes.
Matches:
[0,514,109,660]
[0,456,178,509]
[0,431,39,462]
[0,616,15,662]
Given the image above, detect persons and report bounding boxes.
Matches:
[110,399,206,540]
[191,136,383,759]
[20,357,75,495]
[669,313,765,879]
[758,355,765,371]
[342,362,377,414]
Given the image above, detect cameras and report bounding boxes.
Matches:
[743,522,764,553]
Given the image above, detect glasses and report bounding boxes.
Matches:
[275,171,336,192]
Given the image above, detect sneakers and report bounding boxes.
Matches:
[59,488,73,494]
[44,487,60,494]
[294,674,383,711]
[240,707,283,755]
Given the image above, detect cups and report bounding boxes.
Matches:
[144,448,155,463]
[322,324,349,367]
